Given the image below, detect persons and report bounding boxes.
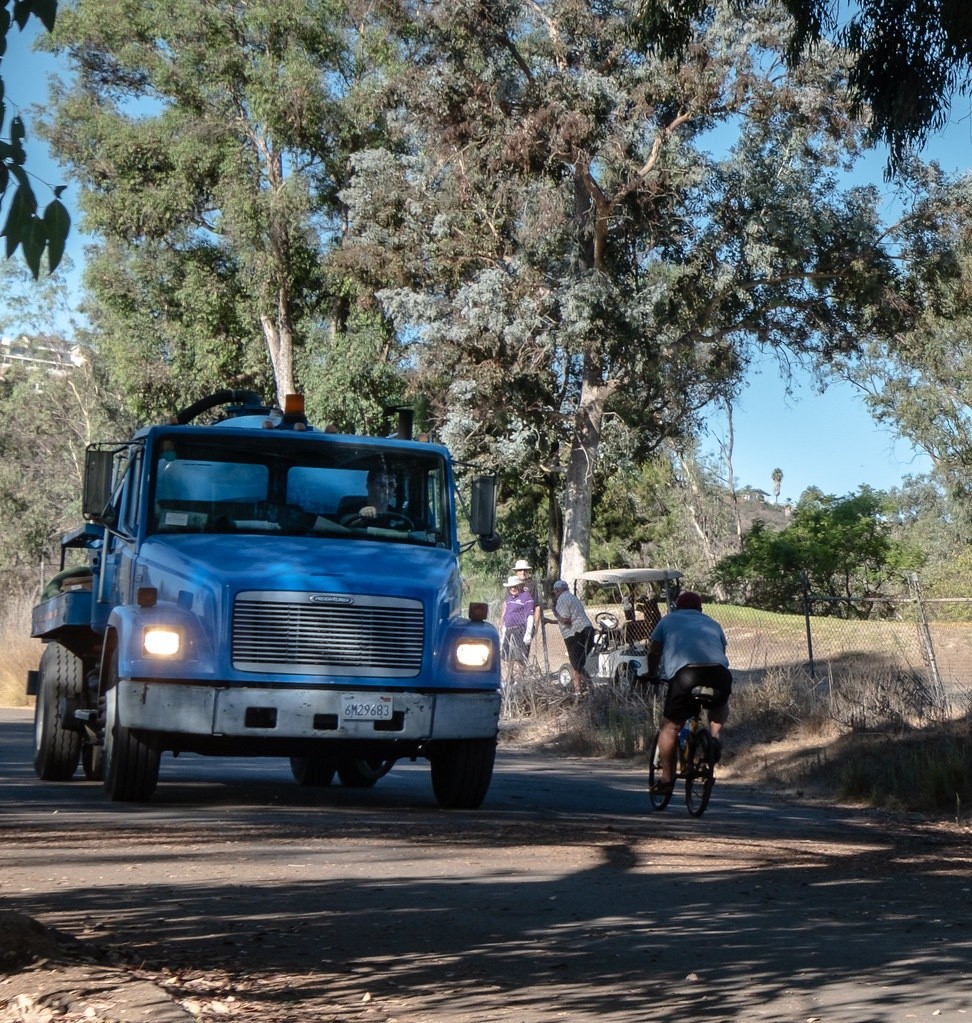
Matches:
[646,590,734,798]
[333,463,422,534]
[496,558,542,697]
[542,579,596,698]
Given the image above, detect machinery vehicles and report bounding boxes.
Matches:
[30,390,503,811]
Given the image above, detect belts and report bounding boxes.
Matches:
[508,625,520,630]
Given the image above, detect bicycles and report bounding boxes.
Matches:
[626,660,722,817]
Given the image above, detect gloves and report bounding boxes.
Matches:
[522,615,534,645]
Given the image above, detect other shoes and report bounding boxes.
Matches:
[652,778,672,794]
[704,739,721,760]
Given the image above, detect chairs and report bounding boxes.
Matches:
[335,496,368,522]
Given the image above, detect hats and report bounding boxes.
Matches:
[676,593,701,610]
[551,581,569,592]
[503,575,525,587]
[512,560,533,570]
